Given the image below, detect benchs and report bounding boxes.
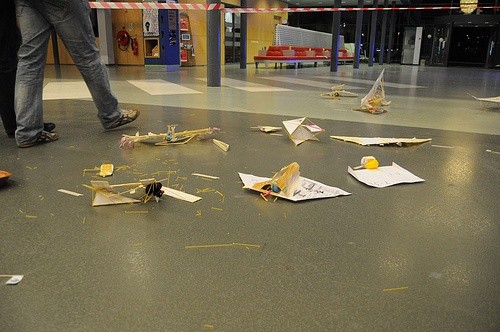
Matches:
[253,24,355,69]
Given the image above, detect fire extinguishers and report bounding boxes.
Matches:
[131,37,140,57]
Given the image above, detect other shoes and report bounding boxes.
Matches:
[8,122,56,139]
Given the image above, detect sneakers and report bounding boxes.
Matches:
[107,108,140,130]
[17,130,58,147]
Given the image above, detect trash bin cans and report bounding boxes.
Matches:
[421,58,426,66]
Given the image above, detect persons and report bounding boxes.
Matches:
[0,0,56,138]
[13,0,142,148]
